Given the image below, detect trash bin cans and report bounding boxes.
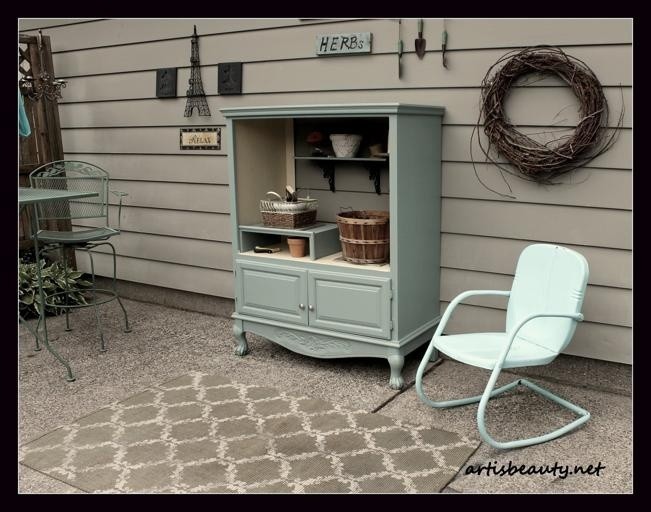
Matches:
[398,39,403,80]
[442,31,447,67]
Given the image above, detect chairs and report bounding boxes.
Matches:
[414,241,593,454]
[28,158,132,353]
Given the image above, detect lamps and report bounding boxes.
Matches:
[20,27,71,105]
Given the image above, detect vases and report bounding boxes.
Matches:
[288,237,307,259]
[328,131,362,160]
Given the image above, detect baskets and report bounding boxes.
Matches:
[260,190,317,229]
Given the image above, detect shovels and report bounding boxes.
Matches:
[414,19,426,60]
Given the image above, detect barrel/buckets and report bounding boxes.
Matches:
[336,211,389,263]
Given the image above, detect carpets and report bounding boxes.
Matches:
[21,371,483,494]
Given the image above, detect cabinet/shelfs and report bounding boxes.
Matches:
[217,100,446,395]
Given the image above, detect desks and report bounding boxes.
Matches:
[18,183,101,384]
